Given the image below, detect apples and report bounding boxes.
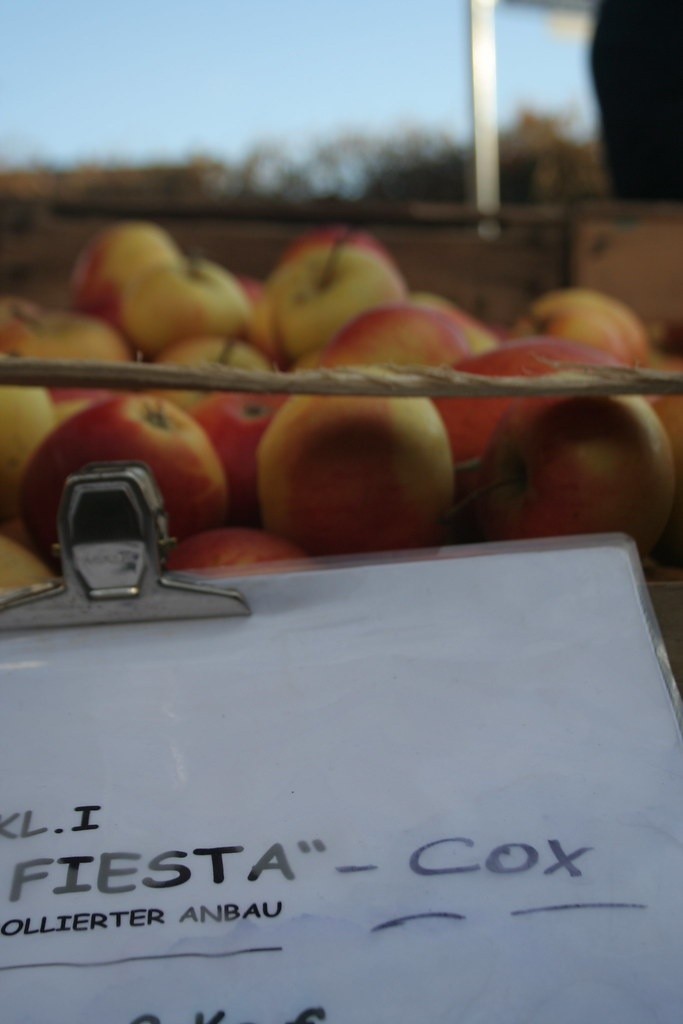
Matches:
[0,220,683,598]
